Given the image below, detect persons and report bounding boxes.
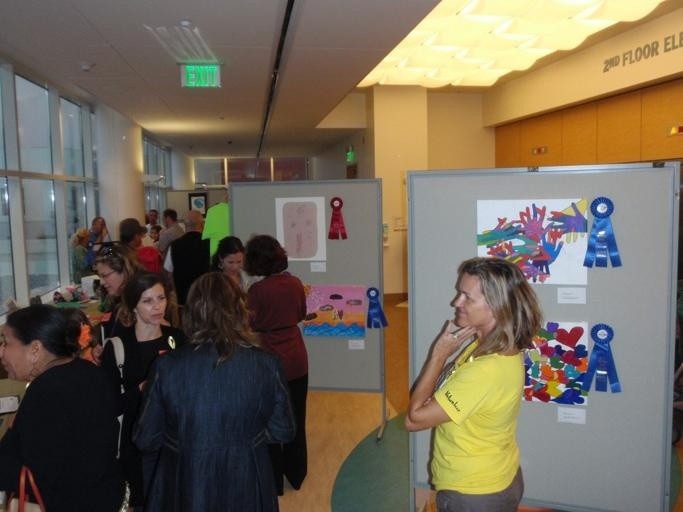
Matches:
[406,258,542,512]
[0,209,307,512]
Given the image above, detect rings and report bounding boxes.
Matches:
[452,333,459,338]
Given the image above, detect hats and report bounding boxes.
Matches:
[120,218,147,238]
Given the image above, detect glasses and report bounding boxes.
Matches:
[97,246,113,257]
[96,270,116,281]
